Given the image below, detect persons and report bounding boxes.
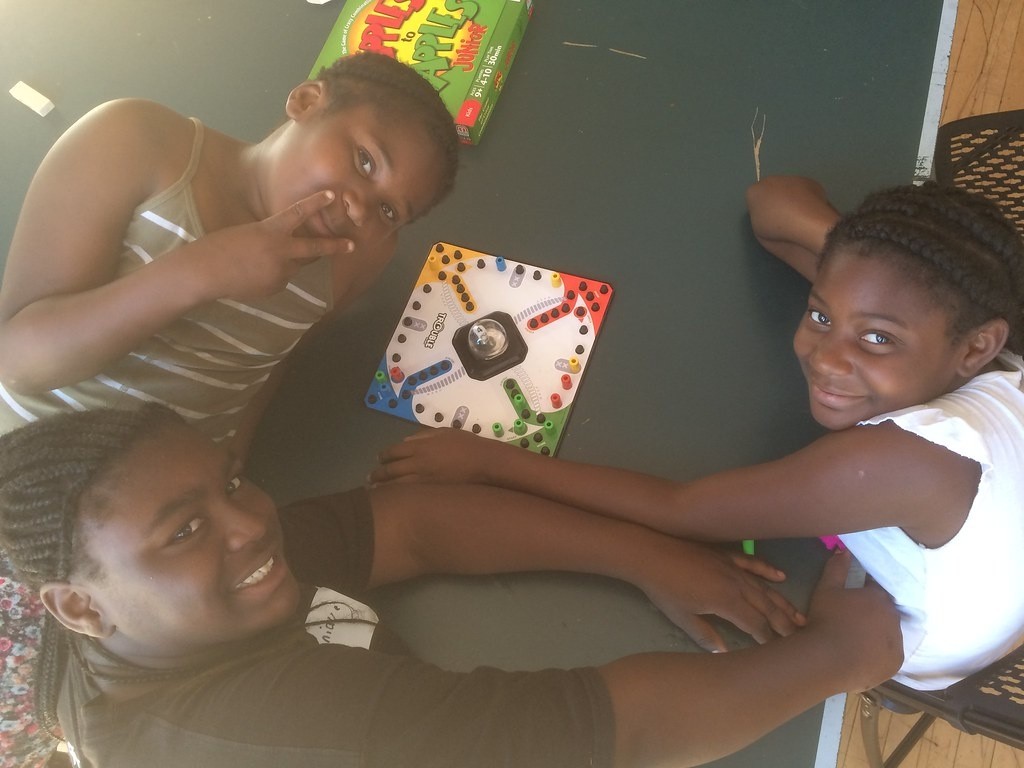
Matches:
[0,46,458,479]
[363,174,1023,693]
[0,405,904,768]
